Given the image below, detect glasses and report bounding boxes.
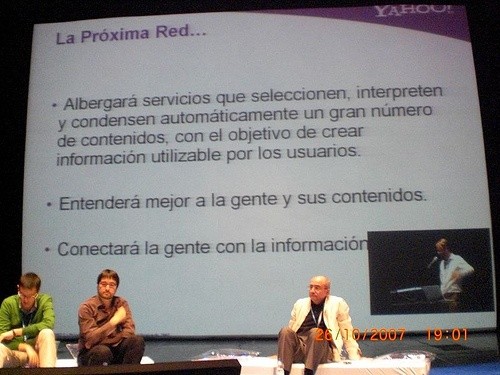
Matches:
[99,281,117,288]
[18,291,38,300]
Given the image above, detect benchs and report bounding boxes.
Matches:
[28,356,154,367]
[199,353,434,375]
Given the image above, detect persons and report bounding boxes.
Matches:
[435,239,474,312]
[76,270,146,367]
[277,276,361,375]
[0,272,57,369]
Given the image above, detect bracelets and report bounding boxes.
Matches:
[12,329,16,337]
